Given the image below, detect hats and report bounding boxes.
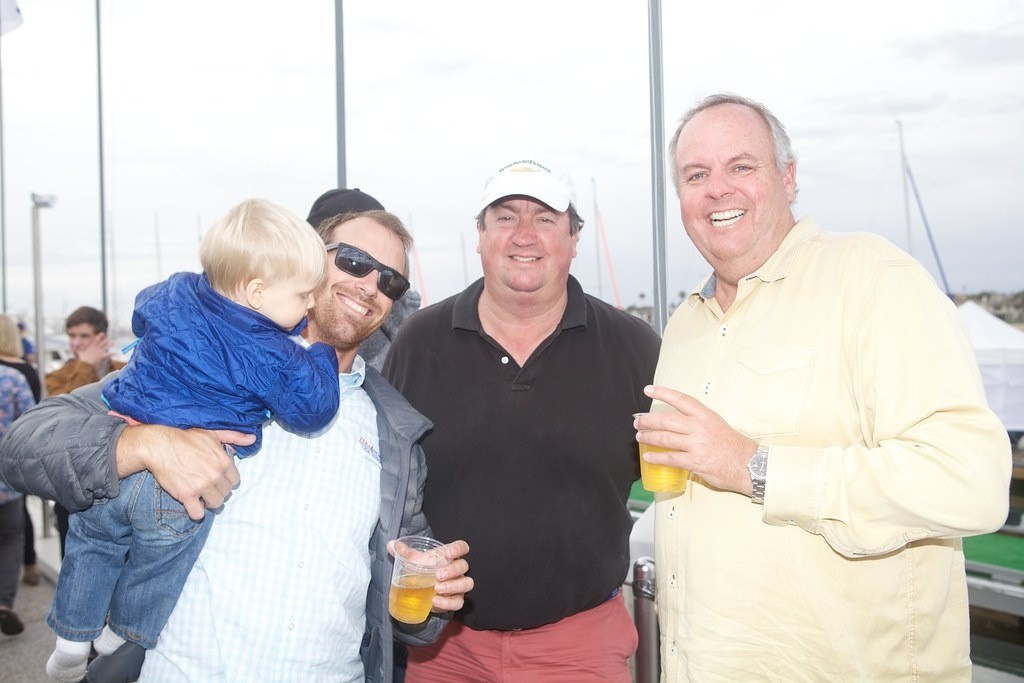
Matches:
[306,188,385,230]
[475,155,585,228]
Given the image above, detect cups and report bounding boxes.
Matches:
[388,536,453,624]
[632,413,689,494]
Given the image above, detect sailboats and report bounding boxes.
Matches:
[891,119,1024,454]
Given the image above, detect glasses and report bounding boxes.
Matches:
[325,242,410,302]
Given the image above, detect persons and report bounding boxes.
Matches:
[0,210,474,683]
[45,198,340,683]
[45,306,127,564]
[380,157,662,683]
[307,188,420,683]
[0,315,41,635]
[634,94,1012,683]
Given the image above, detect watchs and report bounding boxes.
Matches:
[747,445,768,504]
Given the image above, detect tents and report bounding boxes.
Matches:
[957,299,1023,432]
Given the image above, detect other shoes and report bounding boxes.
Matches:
[0,608,23,636]
[23,564,38,585]
